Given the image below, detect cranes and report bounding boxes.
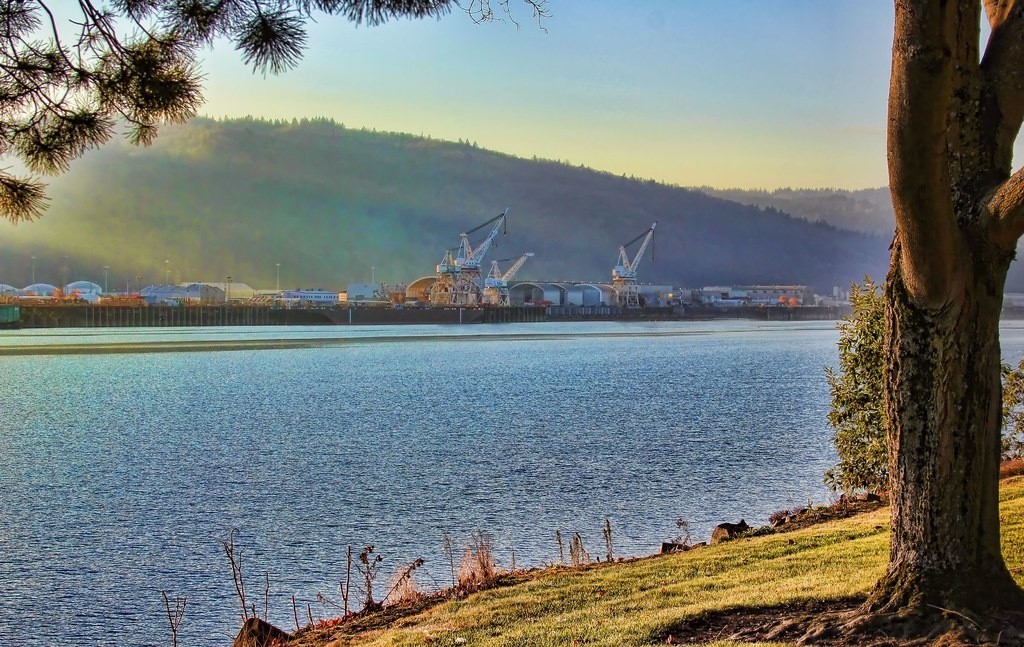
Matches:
[428,206,510,303]
[482,252,536,305]
[611,221,656,307]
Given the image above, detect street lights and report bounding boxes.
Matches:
[103,265,110,296]
[276,262,281,298]
[371,266,375,284]
[30,256,37,296]
